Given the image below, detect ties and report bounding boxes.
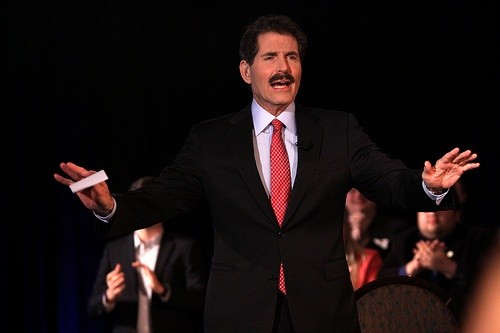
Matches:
[270,120,291,295]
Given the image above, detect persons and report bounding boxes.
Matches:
[342,187,395,292]
[86,177,207,333]
[395,182,474,305]
[54,14,481,333]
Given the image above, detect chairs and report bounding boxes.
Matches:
[354,275,460,333]
